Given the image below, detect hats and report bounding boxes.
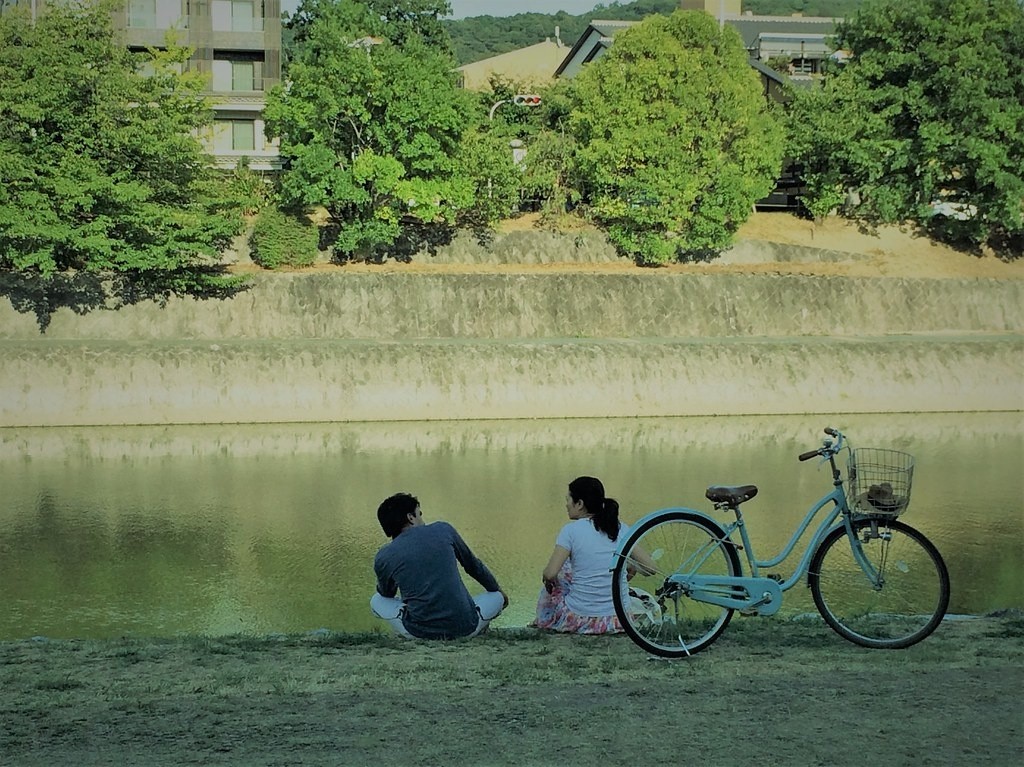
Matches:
[859,482,908,513]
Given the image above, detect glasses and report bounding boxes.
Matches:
[417,511,422,516]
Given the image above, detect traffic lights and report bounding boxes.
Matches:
[514,94,542,106]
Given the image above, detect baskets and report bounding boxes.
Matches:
[847,448,915,516]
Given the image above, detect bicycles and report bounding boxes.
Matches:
[608,427,951,658]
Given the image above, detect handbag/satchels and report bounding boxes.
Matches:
[628,587,663,625]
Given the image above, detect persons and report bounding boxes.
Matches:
[527,476,655,632]
[370,493,508,645]
